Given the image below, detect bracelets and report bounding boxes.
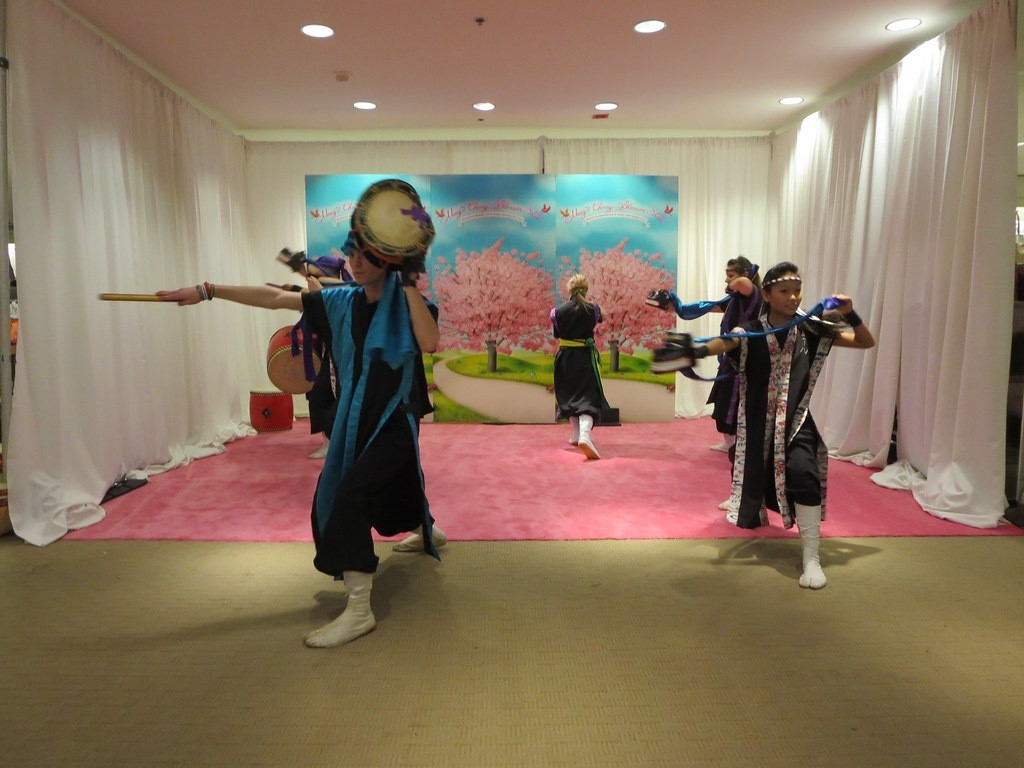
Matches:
[194,282,215,300]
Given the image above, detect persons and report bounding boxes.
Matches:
[550,273,611,459]
[153,178,449,650]
[645,256,876,590]
[266,244,356,459]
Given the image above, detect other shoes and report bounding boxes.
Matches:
[711,441,735,452]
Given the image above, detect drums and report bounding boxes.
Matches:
[265,325,322,395]
[249,389,295,432]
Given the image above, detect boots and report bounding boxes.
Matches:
[796,503,827,588]
[718,499,731,510]
[303,571,376,648]
[569,417,579,444]
[578,414,601,460]
[307,433,330,458]
[393,524,447,552]
